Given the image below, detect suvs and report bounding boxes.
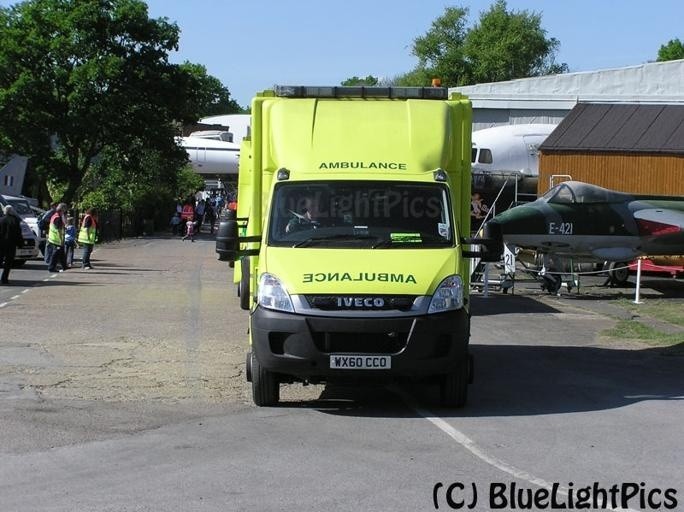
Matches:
[0,194,41,266]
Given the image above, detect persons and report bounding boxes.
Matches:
[0,205,25,284]
[285,193,328,233]
[470,193,484,219]
[169,189,237,242]
[47,203,71,272]
[43,202,57,263]
[64,217,76,267]
[77,207,98,269]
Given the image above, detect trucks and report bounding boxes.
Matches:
[216,86,503,407]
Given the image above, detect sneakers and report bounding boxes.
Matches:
[47,266,73,274]
[82,264,94,270]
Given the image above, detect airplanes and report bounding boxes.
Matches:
[471,123,560,193]
[479,181,684,292]
[173,136,240,173]
[0,156,40,206]
